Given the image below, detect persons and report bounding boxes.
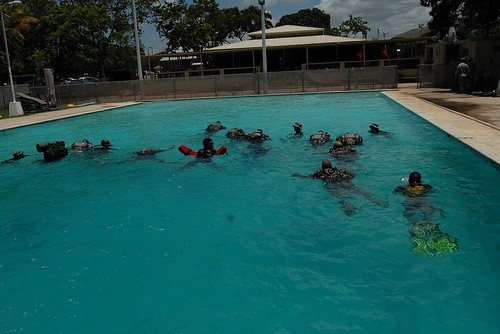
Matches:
[217,121,225,131]
[9,150,30,160]
[293,121,303,134]
[328,141,355,157]
[248,128,271,141]
[368,122,380,133]
[94,138,116,150]
[393,171,433,191]
[196,137,216,158]
[450,56,500,94]
[312,159,354,178]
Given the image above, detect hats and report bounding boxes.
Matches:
[293,122,302,129]
[369,123,379,129]
